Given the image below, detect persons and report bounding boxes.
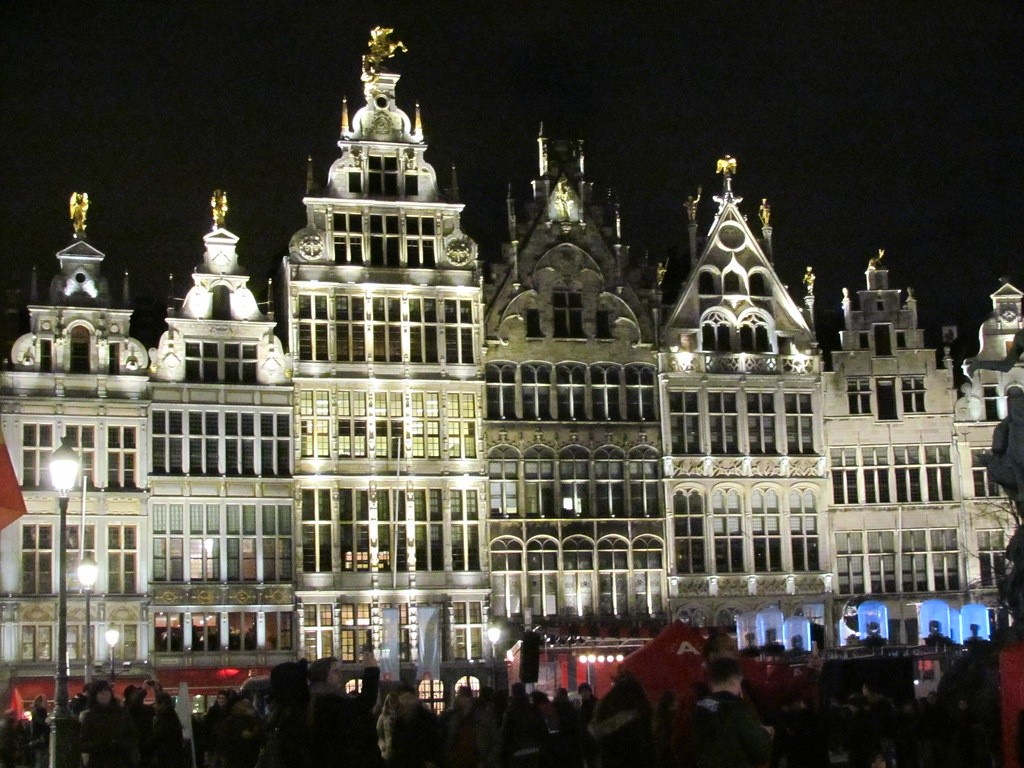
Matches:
[0,621,1024,768]
[990,386,1024,525]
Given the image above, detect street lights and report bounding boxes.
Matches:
[104,621,121,677]
[78,553,99,687]
[42,437,85,768]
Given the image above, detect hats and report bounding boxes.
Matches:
[88,679,108,696]
[123,685,141,704]
[306,657,337,684]
[225,693,244,710]
